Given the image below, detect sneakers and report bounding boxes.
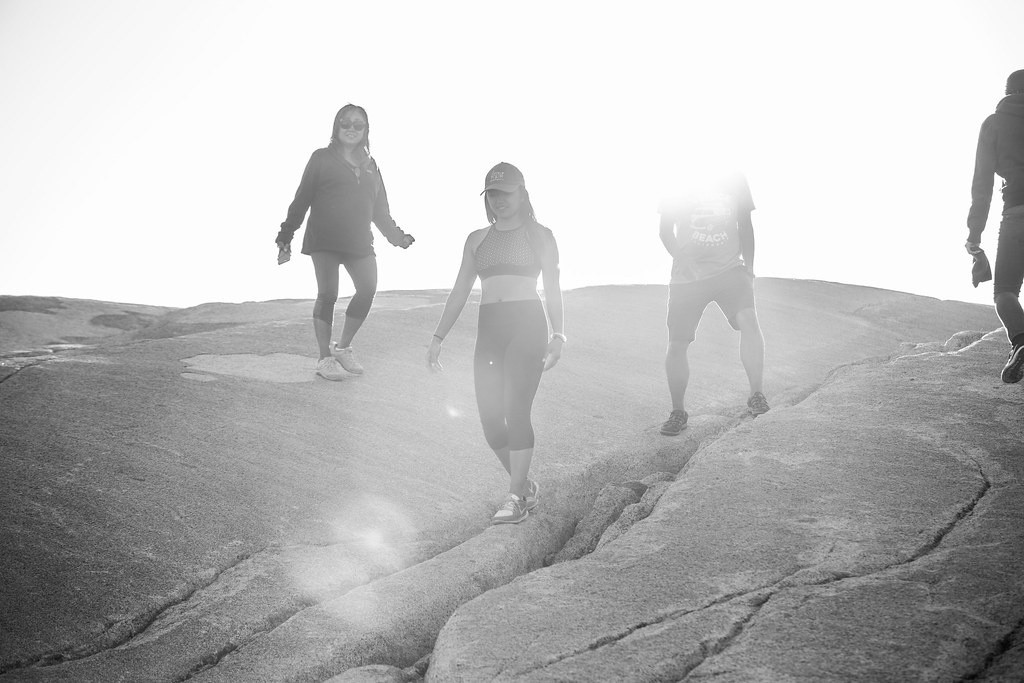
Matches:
[660,409,689,434]
[523,476,539,510]
[491,493,529,524]
[315,358,345,381]
[333,344,363,374]
[747,392,770,414]
[1001,341,1024,384]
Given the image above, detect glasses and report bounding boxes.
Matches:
[339,118,366,131]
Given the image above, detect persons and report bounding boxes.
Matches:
[424,162,564,524]
[659,178,771,436]
[965,68,1024,383]
[276,103,416,381]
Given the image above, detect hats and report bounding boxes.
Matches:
[480,162,524,196]
[1006,70,1024,94]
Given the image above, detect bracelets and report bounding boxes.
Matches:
[432,334,444,341]
[551,332,567,344]
